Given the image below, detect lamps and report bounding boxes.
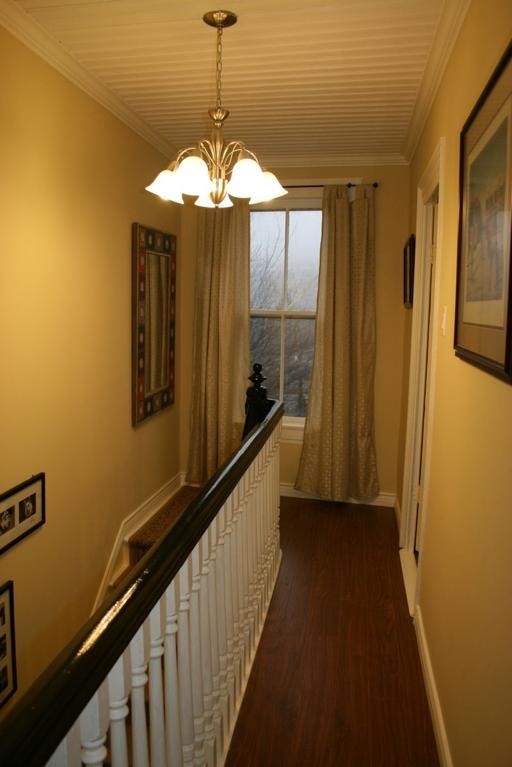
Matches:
[143,9,289,211]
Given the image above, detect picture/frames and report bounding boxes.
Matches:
[404,40,512,384]
[130,221,176,425]
[1,470,46,707]
